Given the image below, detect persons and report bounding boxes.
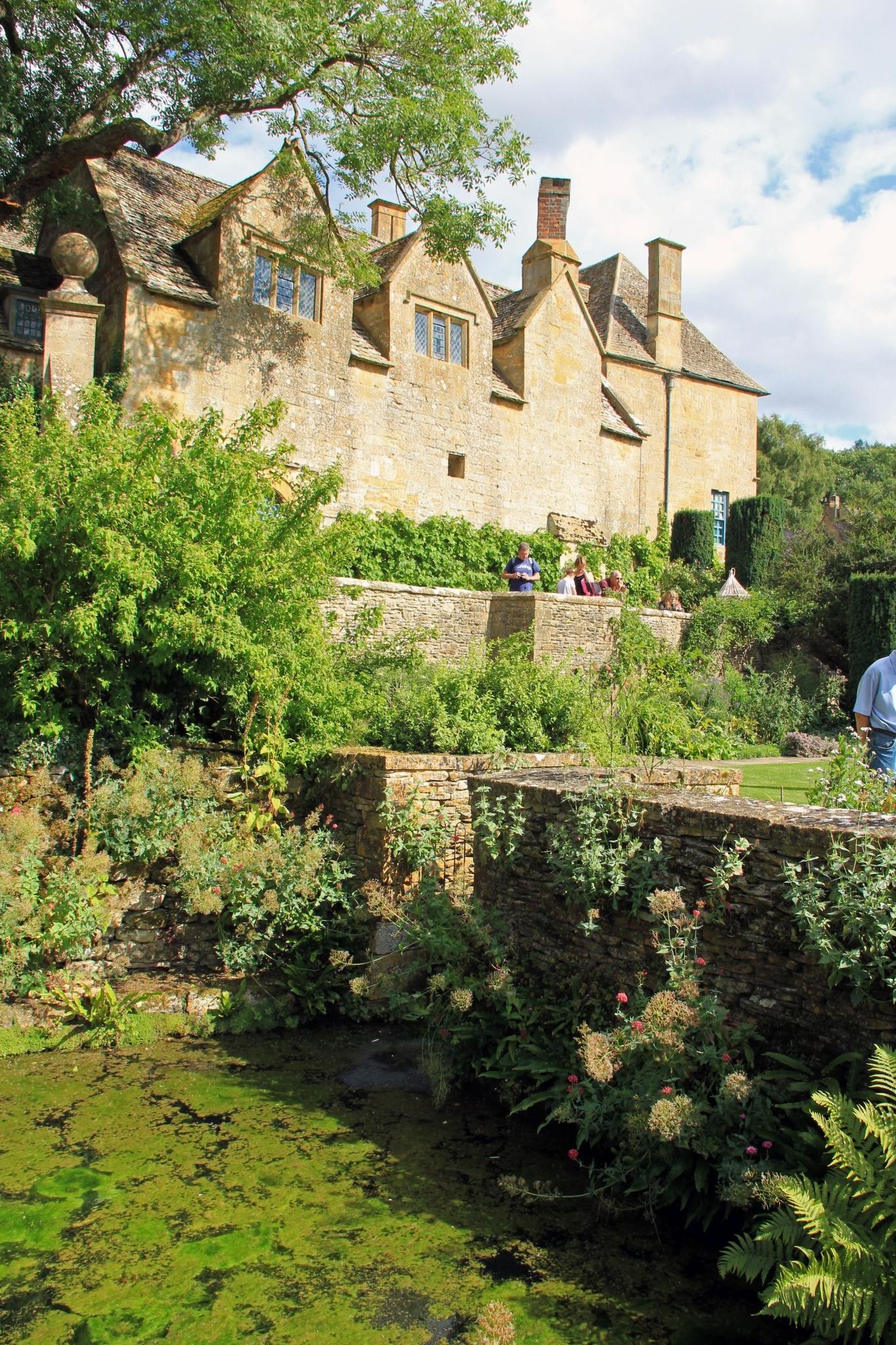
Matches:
[598,570,627,596]
[853,649,896,790]
[556,555,594,596]
[657,589,685,612]
[501,542,541,592]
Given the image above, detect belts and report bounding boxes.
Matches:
[874,728,896,738]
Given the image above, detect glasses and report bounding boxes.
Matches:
[614,577,622,580]
